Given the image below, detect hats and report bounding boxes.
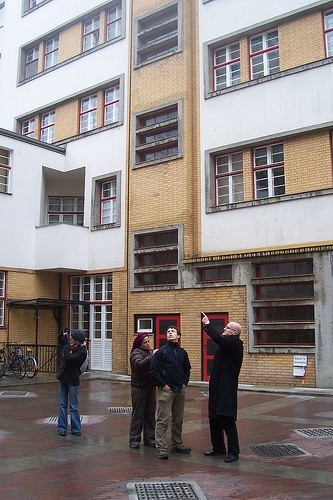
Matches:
[71,330,85,343]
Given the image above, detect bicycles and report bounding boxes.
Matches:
[0,341,38,380]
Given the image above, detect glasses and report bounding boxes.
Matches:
[225,325,236,331]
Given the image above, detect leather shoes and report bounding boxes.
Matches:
[204,450,226,456]
[225,454,239,462]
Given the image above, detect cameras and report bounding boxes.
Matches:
[63,329,68,336]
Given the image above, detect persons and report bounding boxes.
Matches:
[128,332,159,449]
[198,311,245,463]
[148,326,193,459]
[56,329,87,436]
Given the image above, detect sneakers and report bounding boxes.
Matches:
[60,432,66,436]
[172,446,191,453]
[131,442,140,448]
[74,432,81,436]
[157,451,168,459]
[144,443,155,447]
